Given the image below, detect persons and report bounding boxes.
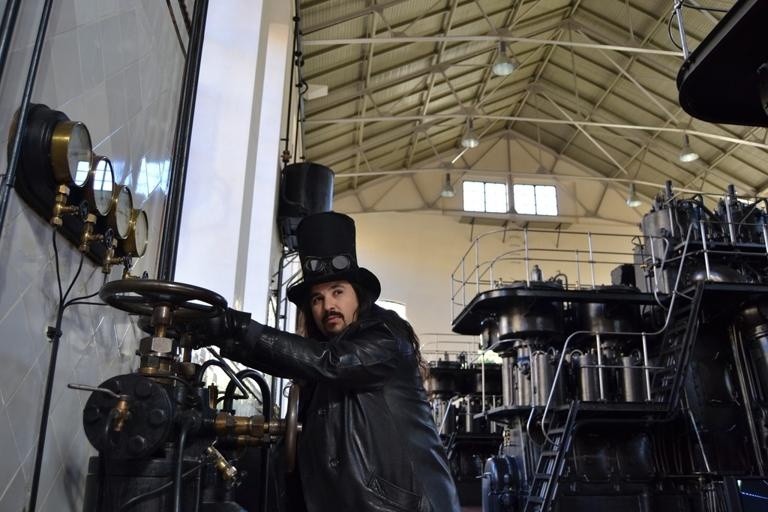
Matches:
[187,279,462,512]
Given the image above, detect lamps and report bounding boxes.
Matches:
[625,181,644,210]
[676,128,701,164]
[458,115,481,149]
[437,169,458,199]
[489,36,515,79]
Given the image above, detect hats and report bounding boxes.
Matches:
[284,211,381,304]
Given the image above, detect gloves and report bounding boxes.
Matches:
[174,307,250,346]
